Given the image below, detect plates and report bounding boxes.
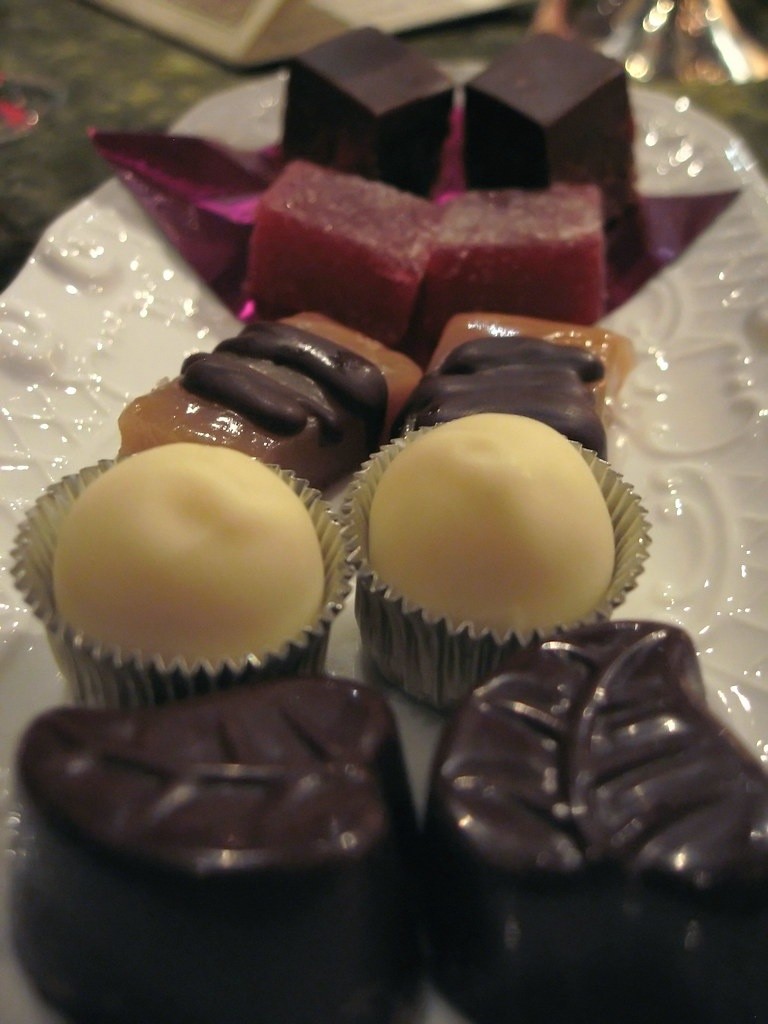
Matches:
[0,64,768,1024]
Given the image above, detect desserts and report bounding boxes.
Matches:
[8,27,768,1024]
